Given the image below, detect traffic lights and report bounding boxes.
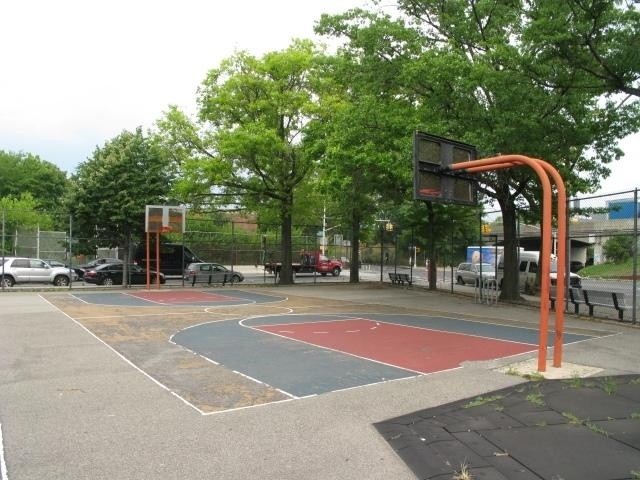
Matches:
[390,224,394,231]
[386,224,389,231]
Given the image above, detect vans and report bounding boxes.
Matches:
[455,244,582,296]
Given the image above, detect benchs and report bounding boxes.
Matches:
[188,272,234,289]
[388,272,415,290]
[549,285,634,323]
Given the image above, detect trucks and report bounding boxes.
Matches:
[263,249,343,280]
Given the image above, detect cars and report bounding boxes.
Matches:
[0,240,245,288]
